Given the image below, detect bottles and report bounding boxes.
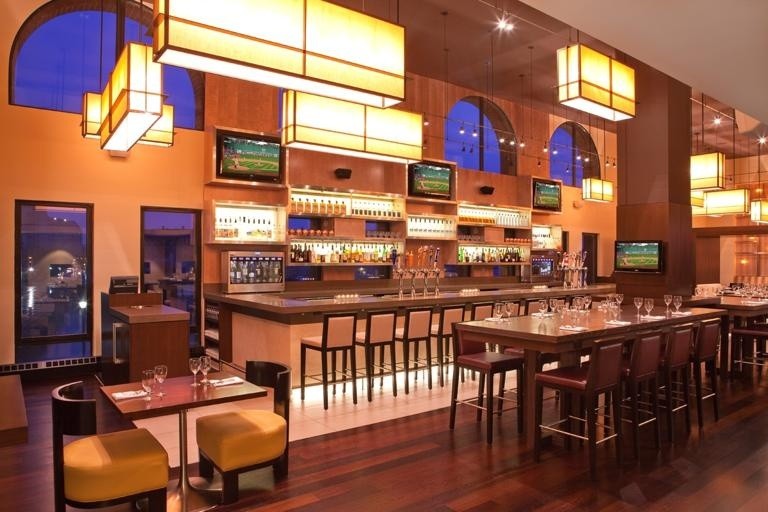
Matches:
[215,197,558,299]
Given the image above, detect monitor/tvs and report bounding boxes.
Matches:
[410,161,452,199]
[615,240,663,271]
[217,130,282,184]
[534,179,560,210]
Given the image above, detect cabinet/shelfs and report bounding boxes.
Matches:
[288,235,406,267]
[107,304,191,382]
[456,203,532,231]
[205,200,287,247]
[447,242,530,265]
[408,214,458,241]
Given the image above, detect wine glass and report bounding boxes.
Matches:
[494,294,683,323]
[538,317,547,334]
[695,280,768,298]
[141,355,211,402]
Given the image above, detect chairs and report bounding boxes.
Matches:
[197,359,292,504]
[50,381,169,511]
